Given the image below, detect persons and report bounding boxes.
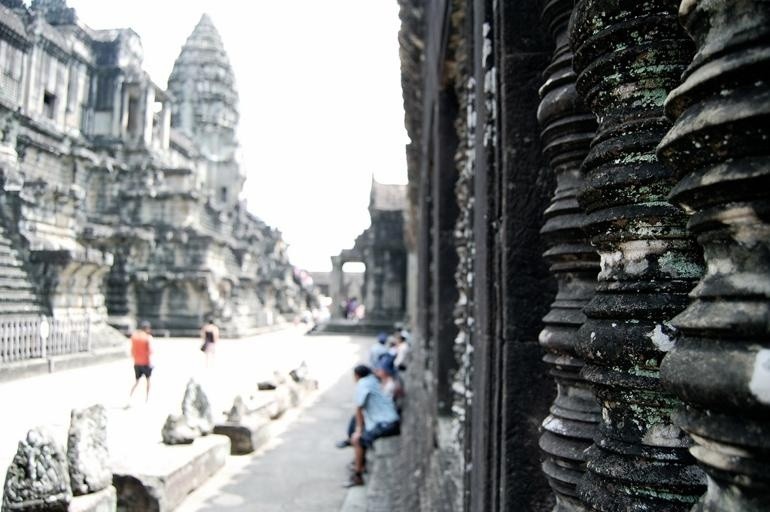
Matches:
[198,315,220,360]
[339,297,358,320]
[338,323,414,487]
[125,319,153,409]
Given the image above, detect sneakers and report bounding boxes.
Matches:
[334,437,367,489]
